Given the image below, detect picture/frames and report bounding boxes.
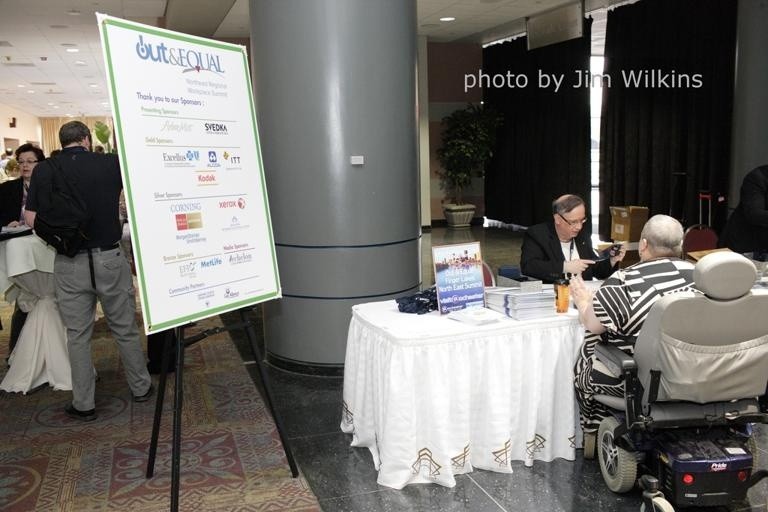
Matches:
[432,241,486,317]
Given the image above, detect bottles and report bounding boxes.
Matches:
[553,278,572,313]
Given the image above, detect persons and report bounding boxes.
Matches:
[0,141,66,369]
[93,145,106,155]
[565,209,715,463]
[518,193,630,279]
[0,147,17,176]
[22,118,157,422]
[442,250,477,271]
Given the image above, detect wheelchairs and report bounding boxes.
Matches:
[580,250,767,512]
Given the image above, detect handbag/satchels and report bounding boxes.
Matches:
[32,159,97,290]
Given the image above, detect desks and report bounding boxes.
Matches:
[339,298,581,491]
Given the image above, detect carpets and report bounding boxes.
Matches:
[0,276,324,512]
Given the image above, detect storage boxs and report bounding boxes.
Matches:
[595,205,649,270]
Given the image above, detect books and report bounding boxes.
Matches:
[483,288,556,319]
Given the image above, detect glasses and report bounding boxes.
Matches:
[17,158,38,166]
[560,217,587,226]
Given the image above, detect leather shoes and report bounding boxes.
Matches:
[64,400,96,422]
[134,383,153,402]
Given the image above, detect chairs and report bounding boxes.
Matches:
[682,224,721,258]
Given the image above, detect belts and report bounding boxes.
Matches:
[100,243,119,251]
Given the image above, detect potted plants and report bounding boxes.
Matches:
[434,101,505,227]
